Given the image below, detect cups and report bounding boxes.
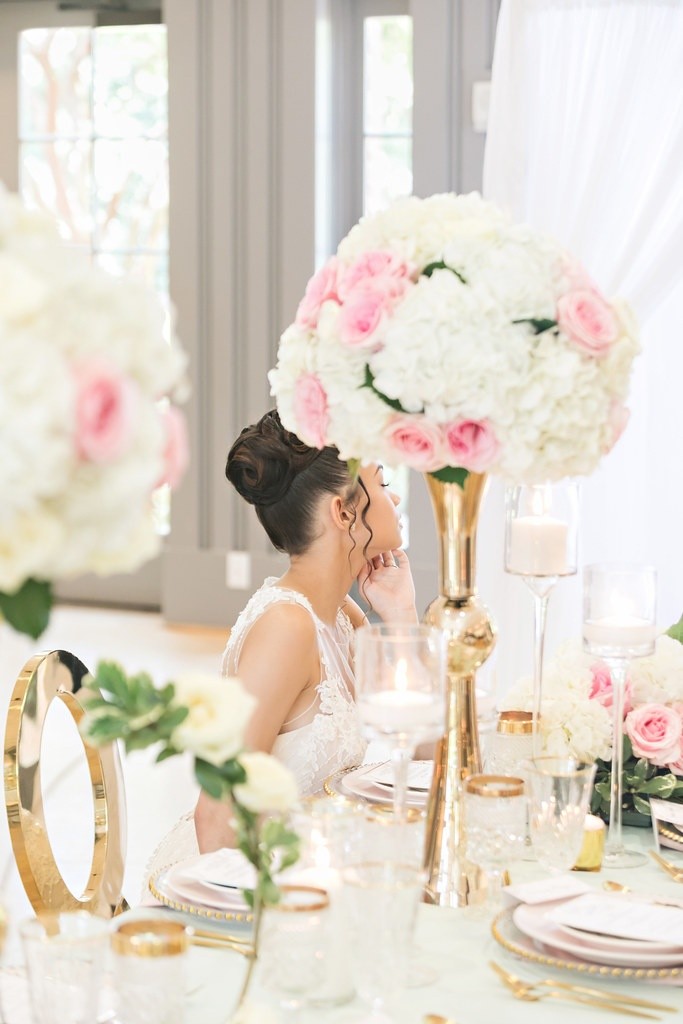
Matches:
[494,711,540,734]
[18,912,193,1024]
[530,757,598,874]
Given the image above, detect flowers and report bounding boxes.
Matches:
[77,661,301,1024]
[498,612,683,826]
[0,192,190,641]
[266,191,640,473]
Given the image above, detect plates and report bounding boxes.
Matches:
[491,882,683,977]
[322,764,435,818]
[149,858,256,921]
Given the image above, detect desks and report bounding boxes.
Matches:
[0,761,683,1024]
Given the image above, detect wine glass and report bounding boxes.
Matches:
[345,621,446,888]
[256,885,331,1023]
[582,561,658,868]
[462,774,525,904]
[504,476,580,758]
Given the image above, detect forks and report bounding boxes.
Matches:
[489,959,660,1020]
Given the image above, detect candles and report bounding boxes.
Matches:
[368,691,436,709]
[585,620,651,644]
[511,518,570,571]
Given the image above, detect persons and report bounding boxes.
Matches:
[195,410,416,854]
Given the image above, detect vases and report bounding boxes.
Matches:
[418,470,490,908]
